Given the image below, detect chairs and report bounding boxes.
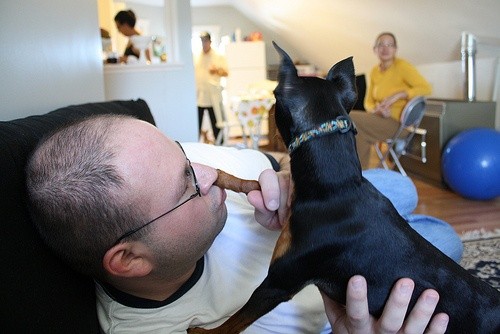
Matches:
[373,97,427,175]
[214,90,277,150]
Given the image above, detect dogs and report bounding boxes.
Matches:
[186,40,500,334]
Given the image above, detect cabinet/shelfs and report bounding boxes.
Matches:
[400,99,496,181]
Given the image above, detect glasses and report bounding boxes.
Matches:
[107,140,202,251]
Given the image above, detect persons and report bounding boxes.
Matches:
[24,112,464,334]
[348,33,431,170]
[194,32,228,145]
[115,10,149,64]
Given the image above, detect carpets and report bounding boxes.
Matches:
[458,229,500,291]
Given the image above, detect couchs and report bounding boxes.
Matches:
[0,100,155,334]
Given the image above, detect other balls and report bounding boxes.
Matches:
[442,128,500,200]
